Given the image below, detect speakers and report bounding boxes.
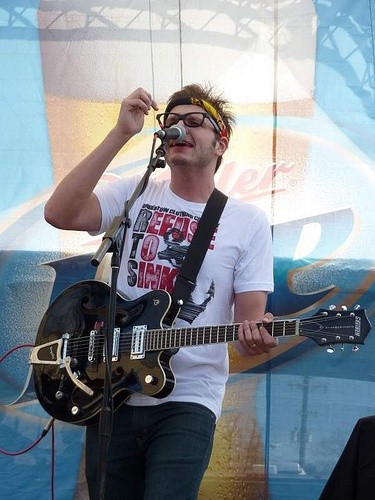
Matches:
[318,415,375,500]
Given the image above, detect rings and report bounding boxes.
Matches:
[249,343,256,348]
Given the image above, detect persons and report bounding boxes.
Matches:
[43,84,280,500]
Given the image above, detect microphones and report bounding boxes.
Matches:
[154,125,186,142]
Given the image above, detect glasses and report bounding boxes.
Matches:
[156,112,223,137]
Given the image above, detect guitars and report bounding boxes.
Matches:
[33,280,371,425]
